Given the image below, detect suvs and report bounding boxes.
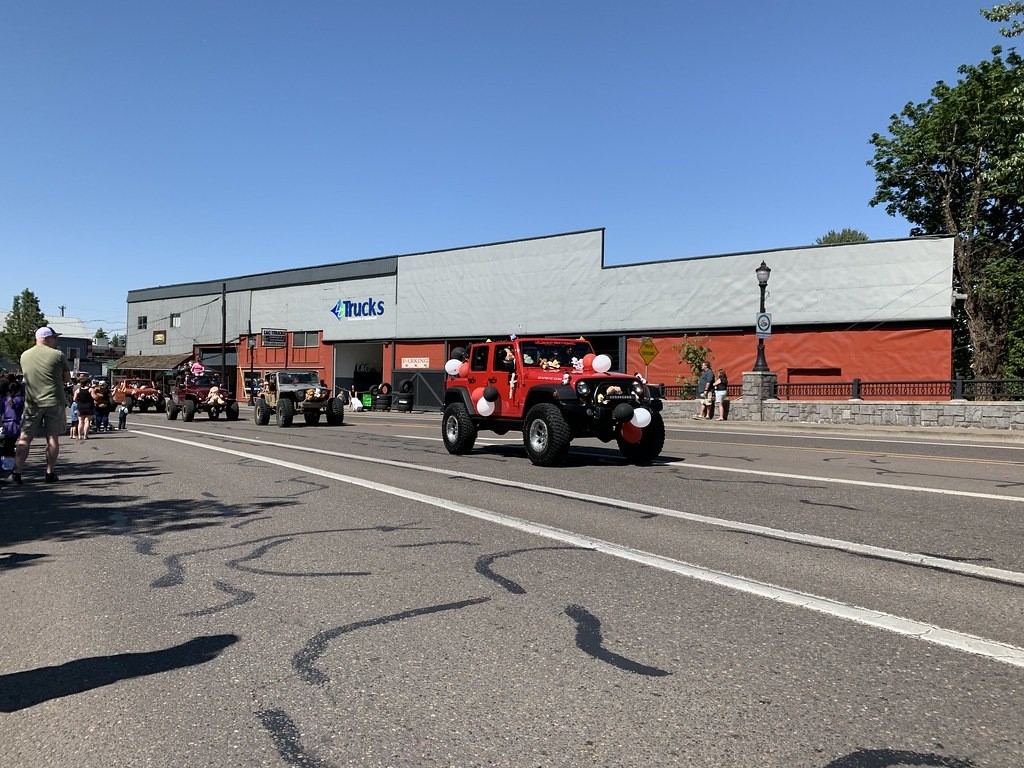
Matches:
[254,370,344,428]
[109,379,166,413]
[441,337,665,466]
[165,373,239,423]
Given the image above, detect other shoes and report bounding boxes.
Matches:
[69,435,89,440]
[11,471,23,482]
[45,472,59,484]
[692,414,704,420]
[716,418,724,421]
[704,417,710,420]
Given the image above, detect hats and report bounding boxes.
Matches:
[97,380,106,386]
[35,326,63,339]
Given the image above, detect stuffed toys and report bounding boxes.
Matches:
[205,385,226,407]
[538,357,561,371]
[571,357,584,371]
[121,380,162,404]
[605,385,622,396]
[503,348,515,361]
[560,371,572,385]
[634,371,648,386]
[305,389,326,403]
[506,372,518,400]
[0,364,131,458]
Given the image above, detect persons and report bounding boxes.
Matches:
[347,385,357,410]
[177,363,190,385]
[12,326,72,486]
[691,362,715,420]
[190,357,206,377]
[336,390,346,404]
[711,368,729,422]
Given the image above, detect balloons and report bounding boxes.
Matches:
[471,386,499,416]
[443,342,472,380]
[615,403,652,444]
[584,351,612,377]
